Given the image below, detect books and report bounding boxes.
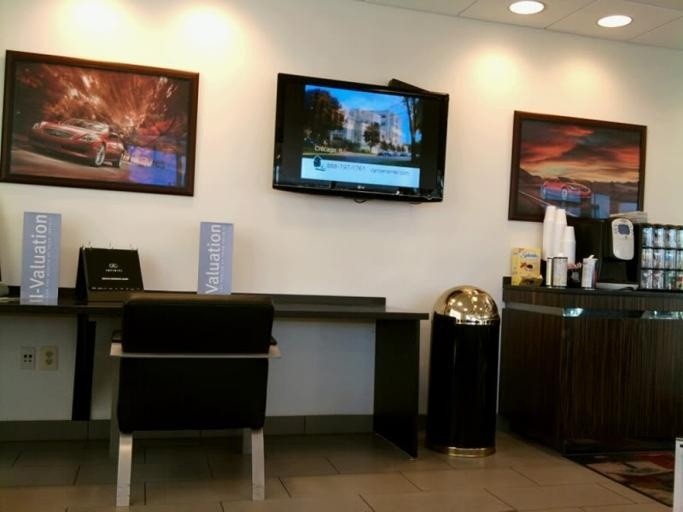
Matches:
[511,246,543,287]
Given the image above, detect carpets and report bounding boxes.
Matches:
[574,448,675,508]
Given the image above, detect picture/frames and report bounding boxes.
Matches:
[0,49,198,198]
[507,110,647,223]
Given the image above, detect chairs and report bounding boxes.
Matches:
[114,296,282,509]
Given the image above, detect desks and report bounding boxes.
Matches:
[0,282,430,463]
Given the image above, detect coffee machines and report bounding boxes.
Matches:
[579,218,640,292]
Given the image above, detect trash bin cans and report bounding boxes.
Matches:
[425,286,500,457]
[674,436,683,512]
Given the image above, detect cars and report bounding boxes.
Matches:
[376,150,411,157]
[540,174,593,202]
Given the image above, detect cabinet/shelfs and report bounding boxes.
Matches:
[502,276,683,461]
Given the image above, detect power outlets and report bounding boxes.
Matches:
[18,345,35,370]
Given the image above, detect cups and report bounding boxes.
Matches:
[581,258,598,292]
[542,206,575,264]
[553,257,567,289]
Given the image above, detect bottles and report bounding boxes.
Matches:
[546,257,552,289]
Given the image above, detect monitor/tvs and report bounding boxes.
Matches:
[272,73,449,202]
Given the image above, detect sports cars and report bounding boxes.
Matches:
[30,115,126,169]
